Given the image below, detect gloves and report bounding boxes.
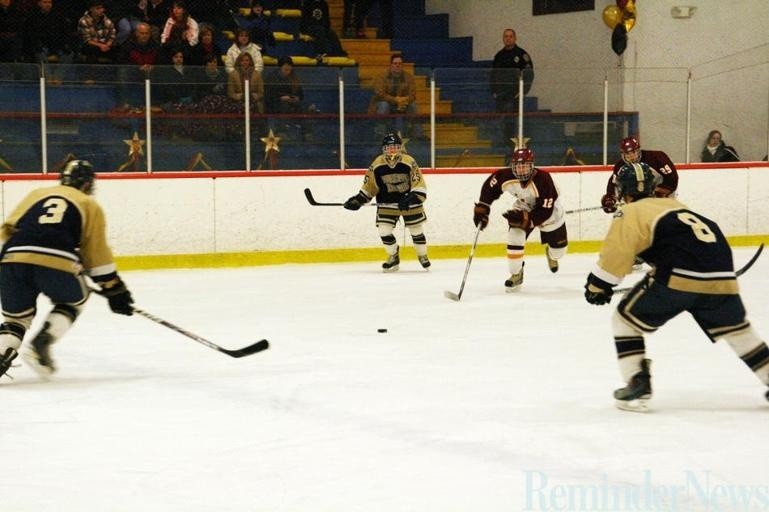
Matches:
[601,195,618,215]
[344,194,368,211]
[583,283,613,305]
[503,209,532,230]
[473,203,491,231]
[395,195,419,211]
[104,282,134,316]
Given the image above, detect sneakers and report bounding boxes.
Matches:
[613,357,653,401]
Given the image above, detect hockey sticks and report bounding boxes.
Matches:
[94,285,268,358]
[305,188,401,209]
[444,219,483,302]
[610,242,765,295]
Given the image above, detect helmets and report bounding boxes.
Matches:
[59,159,93,186]
[620,164,655,197]
[620,137,642,166]
[381,133,402,156]
[510,148,535,181]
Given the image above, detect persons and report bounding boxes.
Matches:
[700,130,728,162]
[490,28,533,143]
[584,163,769,401]
[601,137,678,217]
[345,133,432,268]
[371,54,429,141]
[0,160,136,388]
[473,148,568,288]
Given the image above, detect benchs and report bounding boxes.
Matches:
[1,0,624,171]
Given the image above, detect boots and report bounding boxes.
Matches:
[546,246,559,272]
[505,261,524,287]
[382,251,399,267]
[1,348,19,378]
[418,254,431,268]
[25,333,56,372]
